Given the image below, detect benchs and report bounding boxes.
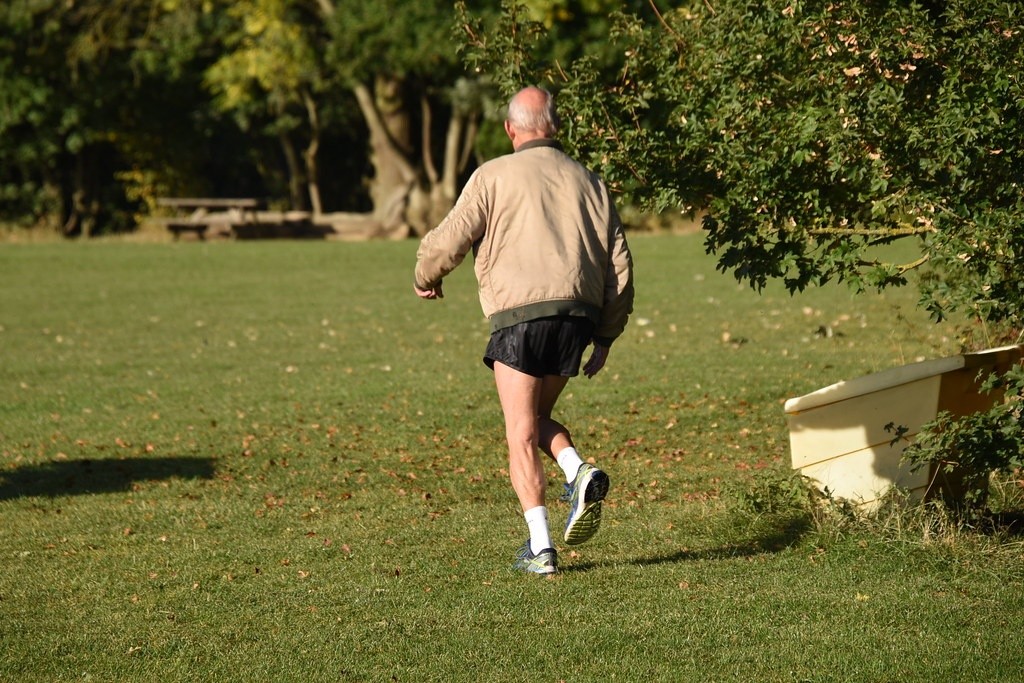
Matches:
[165,217,246,224]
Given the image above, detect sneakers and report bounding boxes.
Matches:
[514,538,558,573]
[564,462,611,545]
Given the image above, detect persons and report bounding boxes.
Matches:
[414,85,634,571]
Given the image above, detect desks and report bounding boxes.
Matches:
[158,197,259,225]
